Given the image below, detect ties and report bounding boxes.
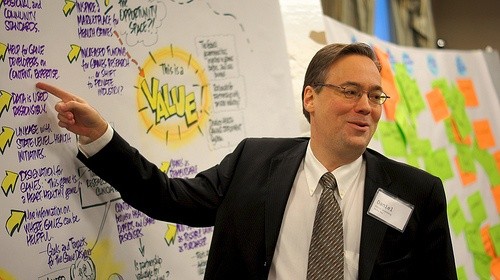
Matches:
[306,172,344,280]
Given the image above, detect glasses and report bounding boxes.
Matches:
[314,82,390,105]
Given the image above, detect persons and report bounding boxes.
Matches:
[34,42,458,280]
